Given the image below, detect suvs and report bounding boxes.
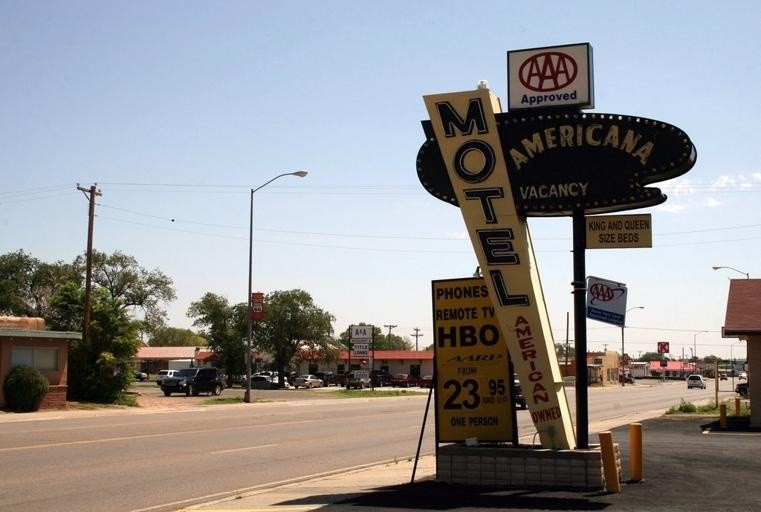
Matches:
[686,375,707,390]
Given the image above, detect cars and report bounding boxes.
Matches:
[157,367,436,397]
[134,370,148,381]
[738,373,747,381]
[720,373,728,380]
[512,371,527,411]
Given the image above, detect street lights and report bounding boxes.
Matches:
[693,330,709,375]
[242,170,310,403]
[621,306,644,387]
[711,265,750,280]
